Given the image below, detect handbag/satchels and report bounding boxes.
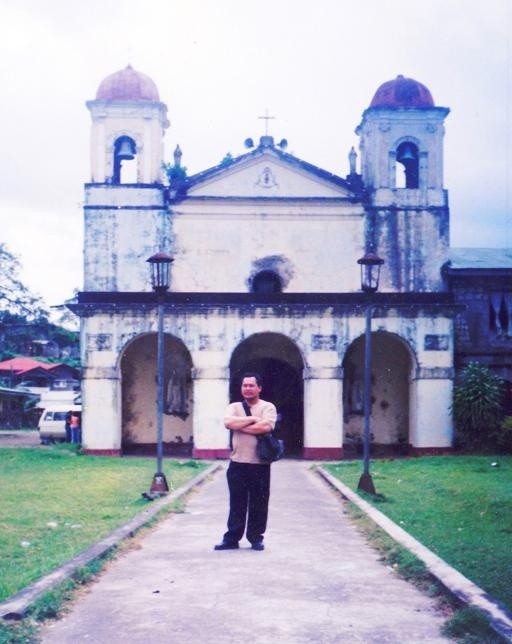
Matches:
[256,434,280,462]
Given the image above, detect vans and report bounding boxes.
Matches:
[37,404,82,445]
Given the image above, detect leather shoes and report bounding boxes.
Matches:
[252,543,263,550]
[215,544,238,550]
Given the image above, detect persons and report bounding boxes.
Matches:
[64,412,70,442]
[70,412,79,444]
[215,373,276,550]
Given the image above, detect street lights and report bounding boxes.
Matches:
[145,247,174,501]
[356,242,385,501]
